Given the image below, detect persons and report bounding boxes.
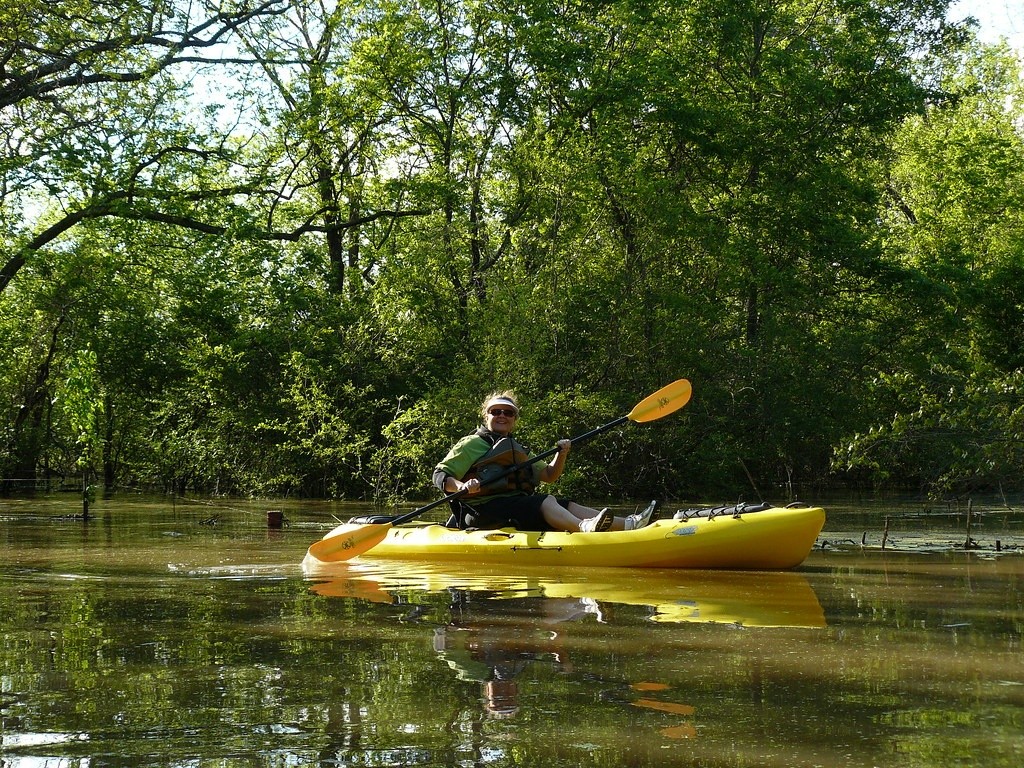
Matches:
[433,393,658,532]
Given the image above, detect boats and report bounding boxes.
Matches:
[302,500,827,604]
[309,550,830,632]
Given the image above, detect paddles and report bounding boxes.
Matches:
[309,578,696,716]
[307,379,693,564]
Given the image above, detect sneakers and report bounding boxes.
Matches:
[582,595,613,623]
[626,499,661,528]
[580,506,614,534]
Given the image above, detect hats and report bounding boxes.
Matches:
[486,399,519,414]
[485,706,518,715]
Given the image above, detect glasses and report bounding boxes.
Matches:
[488,696,517,703]
[487,408,516,418]
